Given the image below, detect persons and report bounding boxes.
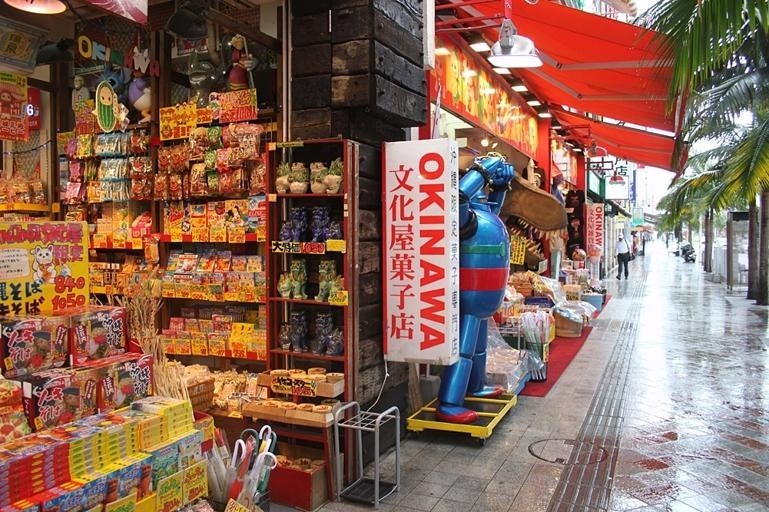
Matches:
[614,233,630,280]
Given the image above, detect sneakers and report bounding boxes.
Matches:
[625,277,628,280]
[616,275,621,280]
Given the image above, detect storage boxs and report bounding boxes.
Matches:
[268,441,345,512]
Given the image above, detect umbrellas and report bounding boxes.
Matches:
[204,425,277,505]
[506,311,549,381]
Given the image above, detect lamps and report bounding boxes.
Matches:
[549,123,607,157]
[584,161,625,184]
[435,0,544,68]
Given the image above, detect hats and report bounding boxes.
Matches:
[618,234,624,239]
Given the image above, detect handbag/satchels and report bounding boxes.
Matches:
[629,253,635,261]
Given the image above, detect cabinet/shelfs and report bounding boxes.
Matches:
[56,122,155,307]
[334,401,402,510]
[157,108,272,408]
[240,138,362,501]
